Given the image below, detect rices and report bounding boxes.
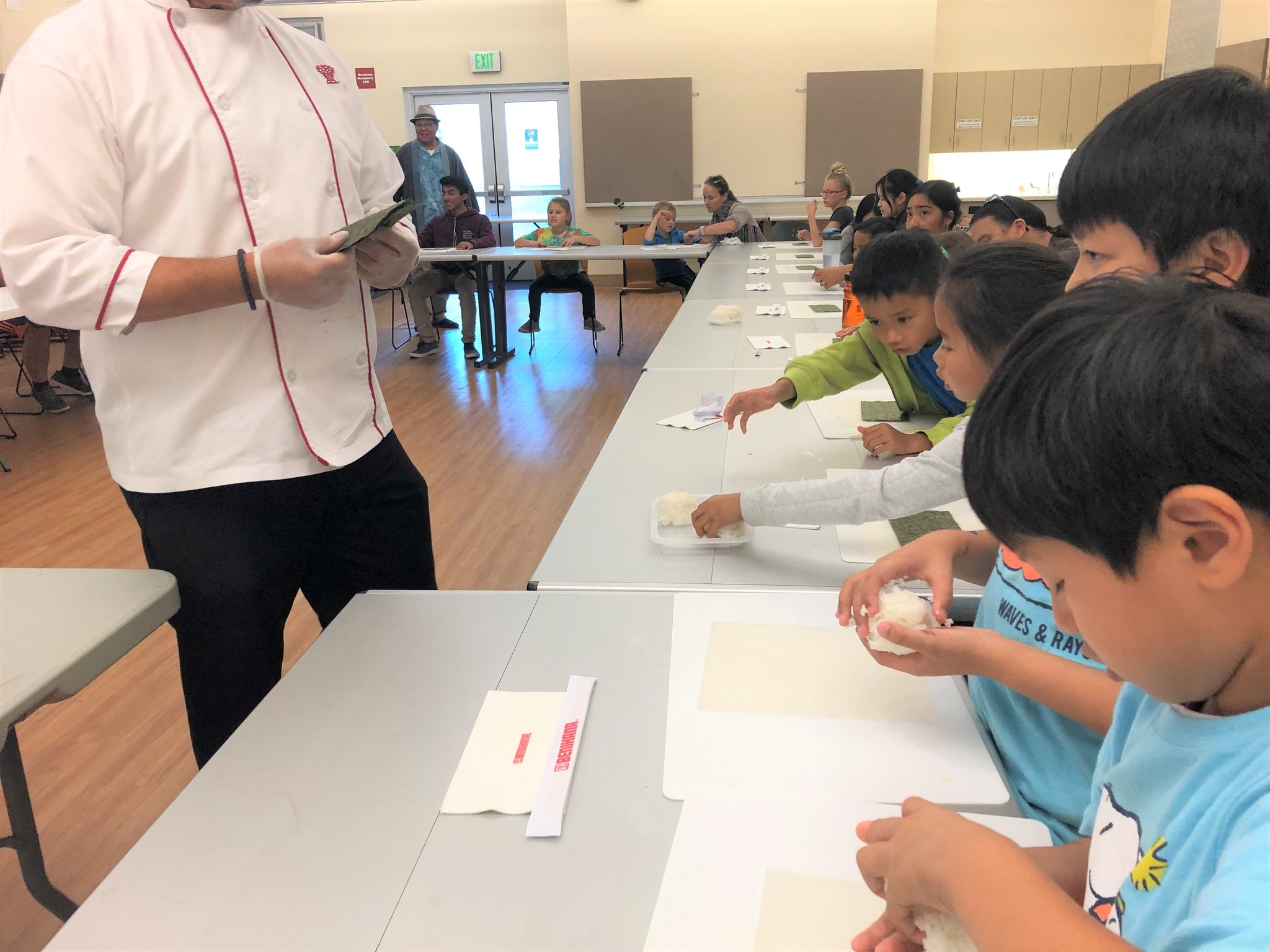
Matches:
[656,492,700,527]
[884,876,978,952]
[703,520,746,538]
[864,577,955,655]
[710,304,741,320]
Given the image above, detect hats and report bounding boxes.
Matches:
[410,105,440,122]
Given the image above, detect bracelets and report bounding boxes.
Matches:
[237,248,257,312]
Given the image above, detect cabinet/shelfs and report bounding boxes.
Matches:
[960,196,1063,229]
[929,64,1162,153]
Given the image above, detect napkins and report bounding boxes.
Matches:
[748,336,792,349]
[758,244,776,248]
[531,249,569,251]
[659,405,744,430]
[745,284,771,290]
[747,268,770,274]
[750,256,769,260]
[447,686,565,816]
[756,306,786,314]
[643,247,678,250]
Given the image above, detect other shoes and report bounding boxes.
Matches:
[431,318,459,328]
[415,326,418,332]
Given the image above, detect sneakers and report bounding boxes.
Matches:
[464,342,480,358]
[584,318,606,331]
[49,370,92,395]
[409,341,439,357]
[518,319,540,333]
[32,385,70,413]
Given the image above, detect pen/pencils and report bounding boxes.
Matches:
[940,245,950,259]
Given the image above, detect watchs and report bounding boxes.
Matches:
[700,225,706,235]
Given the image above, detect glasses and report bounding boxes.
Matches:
[819,190,844,196]
[704,176,728,193]
[416,123,432,129]
[982,195,1029,232]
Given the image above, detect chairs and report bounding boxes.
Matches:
[0,329,93,415]
[617,226,683,356]
[430,287,495,340]
[529,259,598,354]
[371,287,411,349]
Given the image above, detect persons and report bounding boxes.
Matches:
[1,270,93,413]
[514,197,606,333]
[797,164,855,246]
[682,175,767,243]
[643,200,710,304]
[811,166,1080,339]
[393,106,480,333]
[724,229,978,455]
[1,0,437,774]
[853,260,1270,952]
[408,176,497,359]
[691,242,1080,540]
[835,64,1270,847]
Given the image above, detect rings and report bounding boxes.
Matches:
[822,281,825,285]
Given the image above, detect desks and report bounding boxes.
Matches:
[0,217,1060,952]
